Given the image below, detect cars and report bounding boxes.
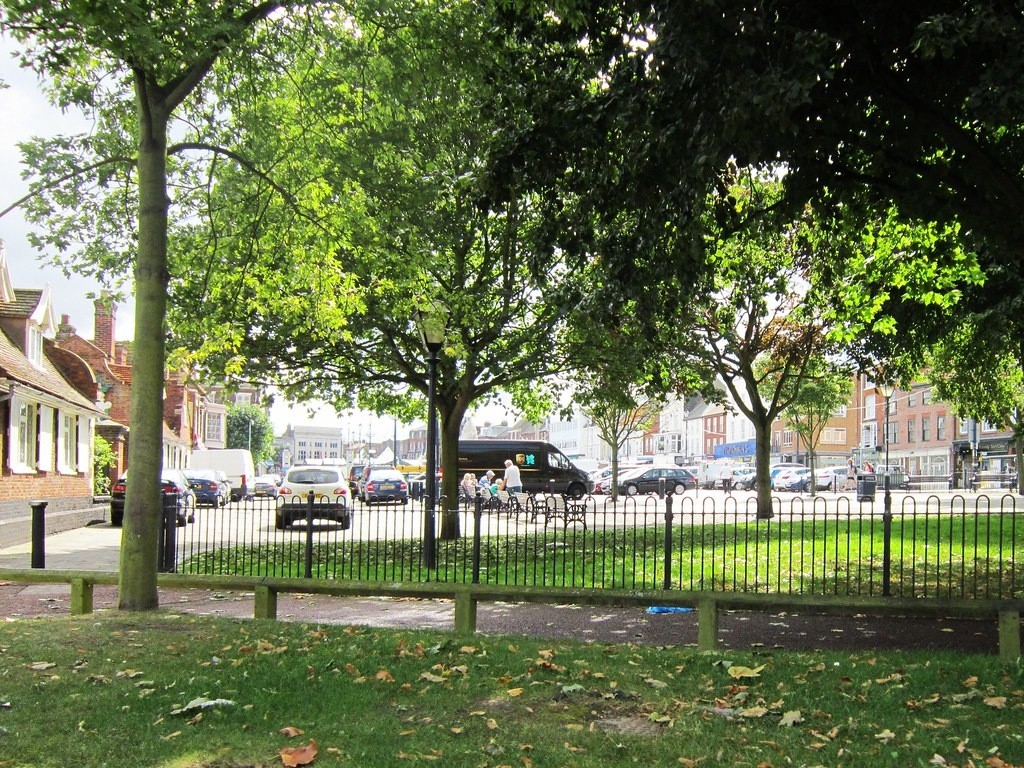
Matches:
[254,473,281,499]
[592,458,864,497]
[107,467,199,526]
[184,468,235,508]
[348,464,440,506]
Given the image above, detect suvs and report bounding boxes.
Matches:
[273,460,355,531]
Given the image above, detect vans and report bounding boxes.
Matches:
[186,448,256,502]
[439,439,596,501]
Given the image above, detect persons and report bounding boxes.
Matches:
[840,458,857,492]
[460,469,503,496]
[499,459,523,494]
[862,460,872,472]
[719,463,734,494]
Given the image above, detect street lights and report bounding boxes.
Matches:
[367,412,375,459]
[248,417,255,451]
[684,409,689,465]
[351,431,356,460]
[356,423,364,464]
[875,377,898,597]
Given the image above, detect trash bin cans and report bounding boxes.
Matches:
[856,473,876,501]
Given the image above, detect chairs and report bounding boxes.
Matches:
[459,485,588,531]
